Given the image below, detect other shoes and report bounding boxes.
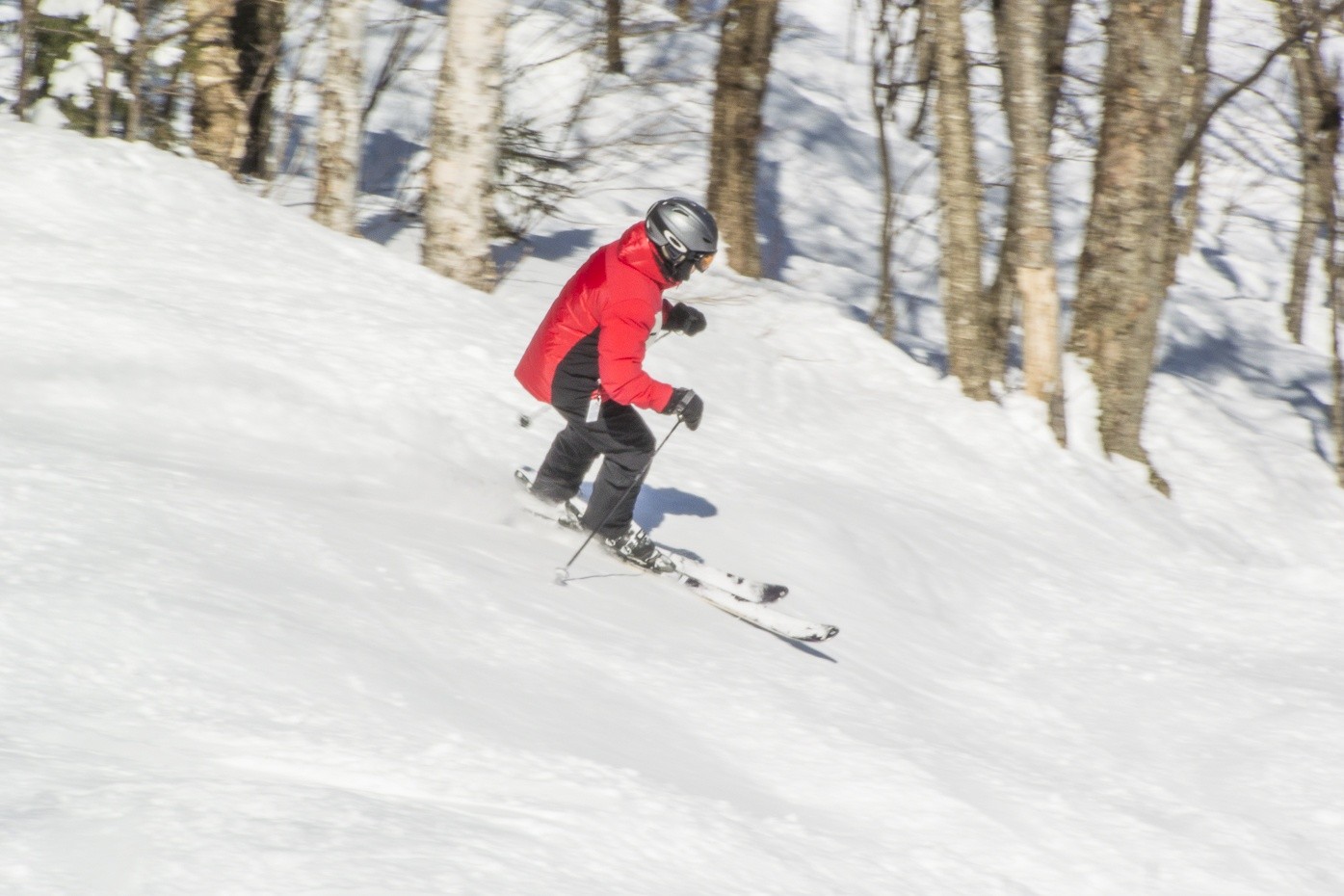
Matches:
[603,530,655,560]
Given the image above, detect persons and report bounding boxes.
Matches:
[511,193,717,565]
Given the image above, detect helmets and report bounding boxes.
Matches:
[645,196,719,282]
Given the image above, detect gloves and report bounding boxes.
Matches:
[661,386,704,431]
[661,302,707,336]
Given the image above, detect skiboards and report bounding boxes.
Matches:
[513,458,839,646]
